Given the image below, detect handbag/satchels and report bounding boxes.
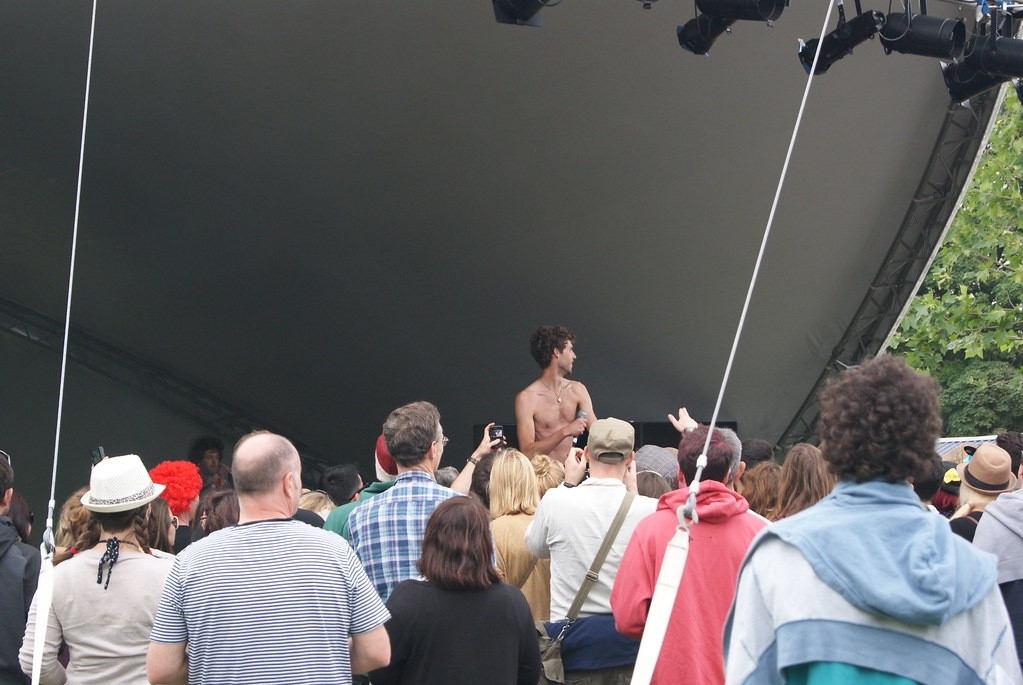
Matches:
[535,623,564,685]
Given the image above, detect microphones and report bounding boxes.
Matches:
[572,411,588,447]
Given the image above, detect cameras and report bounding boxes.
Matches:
[490,426,503,449]
[90,446,105,466]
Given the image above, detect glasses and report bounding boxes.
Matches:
[350,482,369,500]
[27,512,33,525]
[170,516,178,529]
[436,437,449,446]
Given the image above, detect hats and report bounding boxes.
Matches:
[375,434,398,482]
[957,442,1017,494]
[587,417,635,465]
[80,454,166,513]
[940,461,961,494]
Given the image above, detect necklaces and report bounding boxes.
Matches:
[539,378,565,403]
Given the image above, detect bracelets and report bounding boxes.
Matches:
[683,426,696,432]
[561,481,576,488]
[467,456,479,465]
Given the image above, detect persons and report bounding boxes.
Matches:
[0,324,1023,685]
